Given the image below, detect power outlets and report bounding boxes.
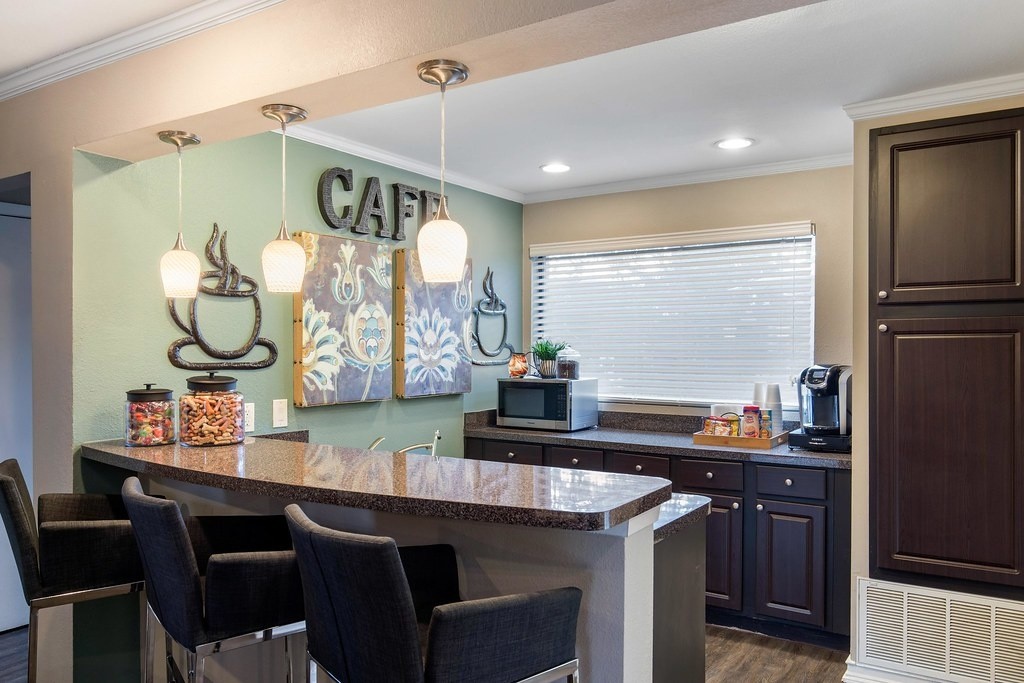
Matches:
[244,402,255,433]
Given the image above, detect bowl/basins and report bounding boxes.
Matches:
[753,383,782,436]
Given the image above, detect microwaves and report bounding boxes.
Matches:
[496,378,598,432]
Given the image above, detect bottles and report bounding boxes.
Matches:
[125,383,174,446]
[179,371,244,447]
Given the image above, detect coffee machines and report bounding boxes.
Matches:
[788,363,852,453]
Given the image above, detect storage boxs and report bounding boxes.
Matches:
[706,405,773,438]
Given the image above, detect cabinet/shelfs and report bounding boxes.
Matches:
[868,104,1024,604]
[464,436,852,653]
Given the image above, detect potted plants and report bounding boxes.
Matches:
[533,337,568,378]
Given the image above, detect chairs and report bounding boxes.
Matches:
[0,457,585,683]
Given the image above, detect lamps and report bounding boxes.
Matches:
[417,59,470,282]
[261,105,308,292]
[158,130,202,299]
